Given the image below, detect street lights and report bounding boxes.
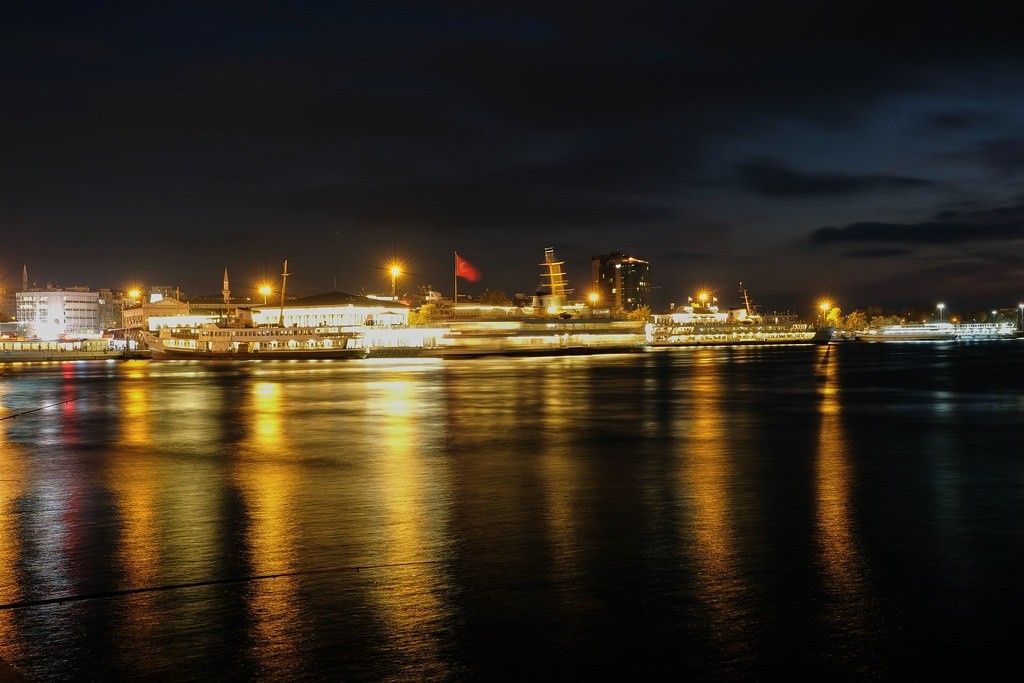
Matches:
[589,292,599,306]
[698,292,707,308]
[1020,304,1024,331]
[261,287,270,304]
[992,311,997,321]
[937,304,945,323]
[819,303,831,321]
[390,267,400,300]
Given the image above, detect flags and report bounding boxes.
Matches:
[455,256,479,282]
[713,296,717,301]
[688,296,692,301]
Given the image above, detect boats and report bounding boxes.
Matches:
[650,285,817,346]
[0,337,125,361]
[439,249,652,360]
[855,326,957,342]
[138,259,371,361]
